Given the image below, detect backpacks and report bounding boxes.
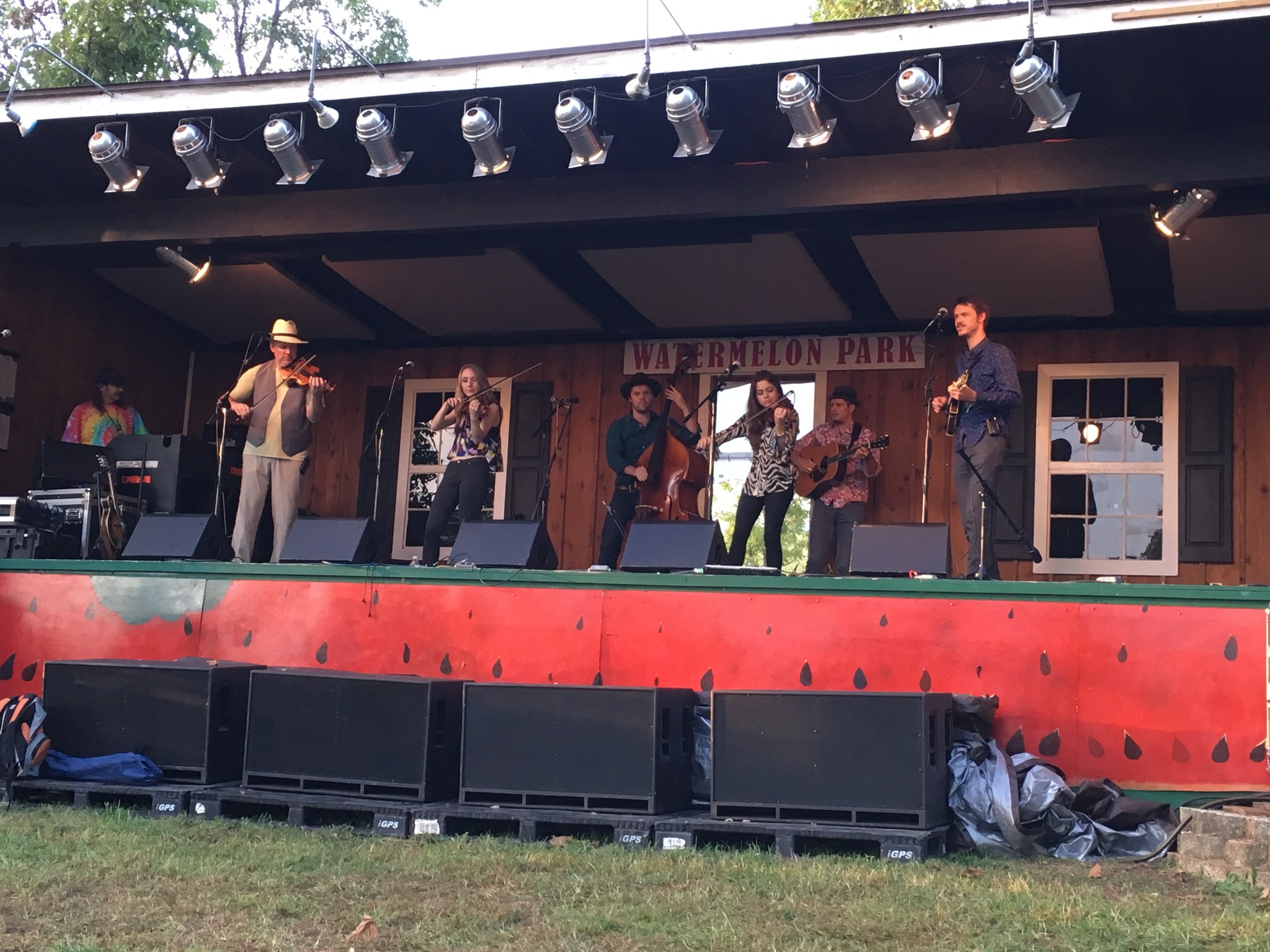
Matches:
[0,691,51,779]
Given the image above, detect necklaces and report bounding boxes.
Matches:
[105,408,124,435]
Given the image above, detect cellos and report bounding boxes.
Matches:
[616,356,708,570]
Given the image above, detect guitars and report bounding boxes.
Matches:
[946,372,968,436]
[794,435,890,500]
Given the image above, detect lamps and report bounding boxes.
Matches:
[1151,188,1219,240]
[775,64,837,148]
[264,109,324,184]
[172,116,232,190]
[1078,421,1103,444]
[461,96,516,177]
[4,42,114,138]
[1134,417,1163,452]
[895,52,960,141]
[555,85,614,168]
[89,121,150,192]
[155,240,212,286]
[665,75,723,158]
[1011,0,1080,132]
[625,0,696,101]
[355,102,414,177]
[307,24,384,129]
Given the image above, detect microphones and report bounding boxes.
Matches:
[399,360,416,372]
[564,398,579,403]
[550,396,569,409]
[928,307,949,326]
[724,361,742,373]
[258,331,274,342]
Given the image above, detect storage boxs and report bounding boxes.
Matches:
[27,486,147,560]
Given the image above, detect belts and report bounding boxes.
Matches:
[616,483,638,493]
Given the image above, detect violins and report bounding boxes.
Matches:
[280,356,334,392]
[461,393,490,418]
[765,397,796,420]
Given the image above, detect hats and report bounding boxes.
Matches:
[620,372,662,399]
[270,318,309,345]
[93,366,131,385]
[828,383,861,407]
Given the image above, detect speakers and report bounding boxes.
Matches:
[617,518,728,574]
[125,511,239,565]
[453,521,559,571]
[849,522,954,581]
[35,439,118,493]
[39,658,260,787]
[457,682,697,814]
[708,686,952,829]
[279,516,386,566]
[244,666,462,804]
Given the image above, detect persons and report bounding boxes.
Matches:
[223,316,328,562]
[696,369,800,578]
[929,296,1025,580]
[416,363,505,568]
[791,384,881,579]
[60,368,151,448]
[597,375,702,571]
